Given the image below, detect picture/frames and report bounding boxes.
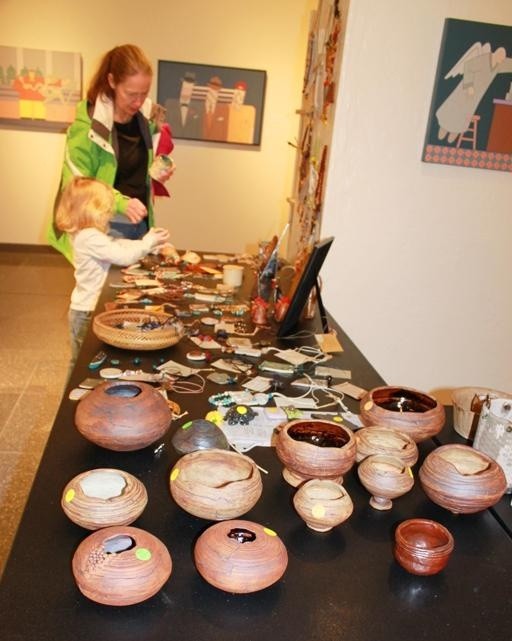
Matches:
[156,60,267,147]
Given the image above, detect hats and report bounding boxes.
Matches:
[207,75,224,90]
[179,71,198,85]
[233,80,248,90]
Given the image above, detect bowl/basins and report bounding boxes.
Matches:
[95,309,185,351]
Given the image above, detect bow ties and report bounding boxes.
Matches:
[179,102,188,108]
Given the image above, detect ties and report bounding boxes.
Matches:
[206,103,213,134]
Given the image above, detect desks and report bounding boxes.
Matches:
[0,248,511,639]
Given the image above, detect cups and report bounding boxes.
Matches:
[394,515,455,577]
[222,265,245,287]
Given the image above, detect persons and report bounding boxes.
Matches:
[55,176,171,393]
[46,44,177,268]
[435,47,512,144]
